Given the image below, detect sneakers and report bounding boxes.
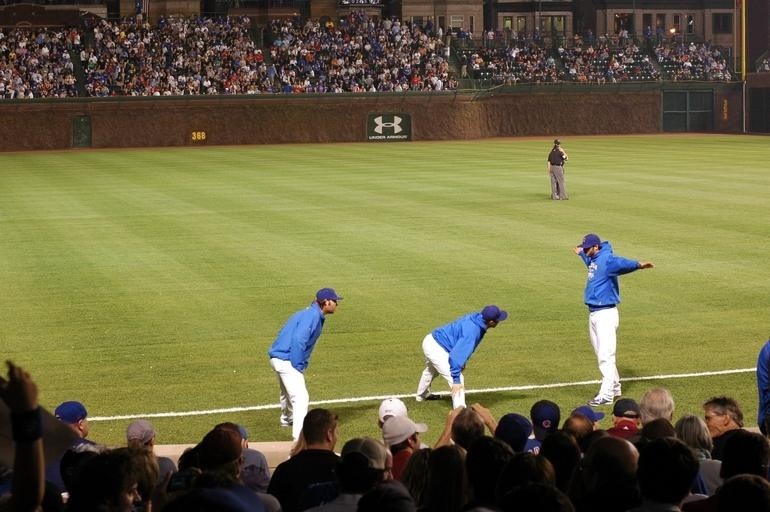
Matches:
[279,414,293,427]
[587,391,622,406]
[427,395,440,400]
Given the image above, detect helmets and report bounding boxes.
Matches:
[530,400,560,436]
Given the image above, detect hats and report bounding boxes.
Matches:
[481,305,507,321]
[201,422,246,462]
[316,287,344,303]
[54,401,87,423]
[341,436,386,471]
[577,234,600,248]
[554,138,560,143]
[494,413,532,447]
[127,420,154,445]
[379,398,427,446]
[571,406,605,422]
[610,398,640,418]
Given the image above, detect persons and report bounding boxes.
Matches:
[266,287,345,442]
[416,303,511,411]
[547,145,570,200]
[551,138,569,195]
[2,1,770,102]
[574,233,654,407]
[2,343,770,512]
